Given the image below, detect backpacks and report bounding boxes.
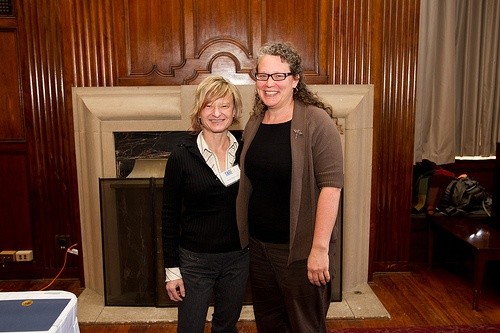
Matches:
[413,159,489,217]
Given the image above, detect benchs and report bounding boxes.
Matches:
[427,216,500,312]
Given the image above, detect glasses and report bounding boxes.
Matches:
[255,73,294,81]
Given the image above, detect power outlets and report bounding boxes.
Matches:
[16,250,33,261]
[0,251,16,262]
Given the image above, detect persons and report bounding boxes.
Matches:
[159,75,251,333]
[235,40,345,333]
